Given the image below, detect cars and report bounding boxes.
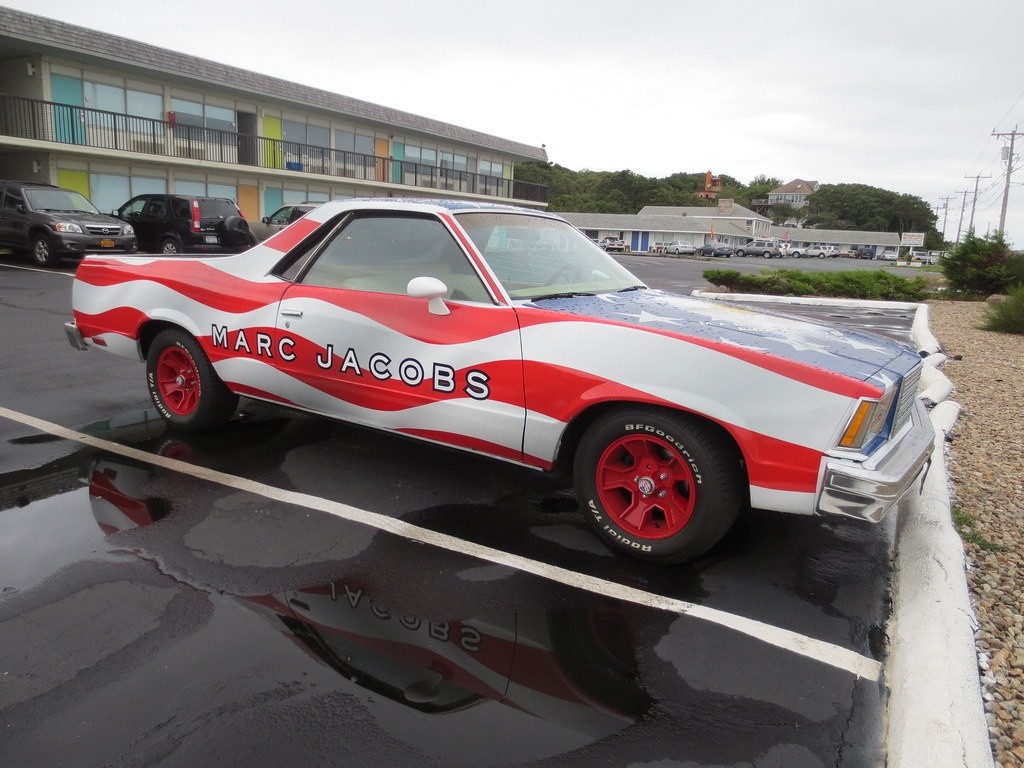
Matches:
[880,250,897,262]
[664,240,696,256]
[249,201,326,248]
[848,250,857,257]
[804,244,828,259]
[599,236,625,252]
[64,196,935,566]
[699,241,733,258]
[911,252,928,262]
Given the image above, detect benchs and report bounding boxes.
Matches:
[342,262,492,303]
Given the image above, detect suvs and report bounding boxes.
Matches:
[0,182,138,267]
[734,240,779,259]
[857,248,873,260]
[820,246,841,258]
[111,194,254,257]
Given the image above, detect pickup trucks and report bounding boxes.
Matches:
[776,243,808,258]
